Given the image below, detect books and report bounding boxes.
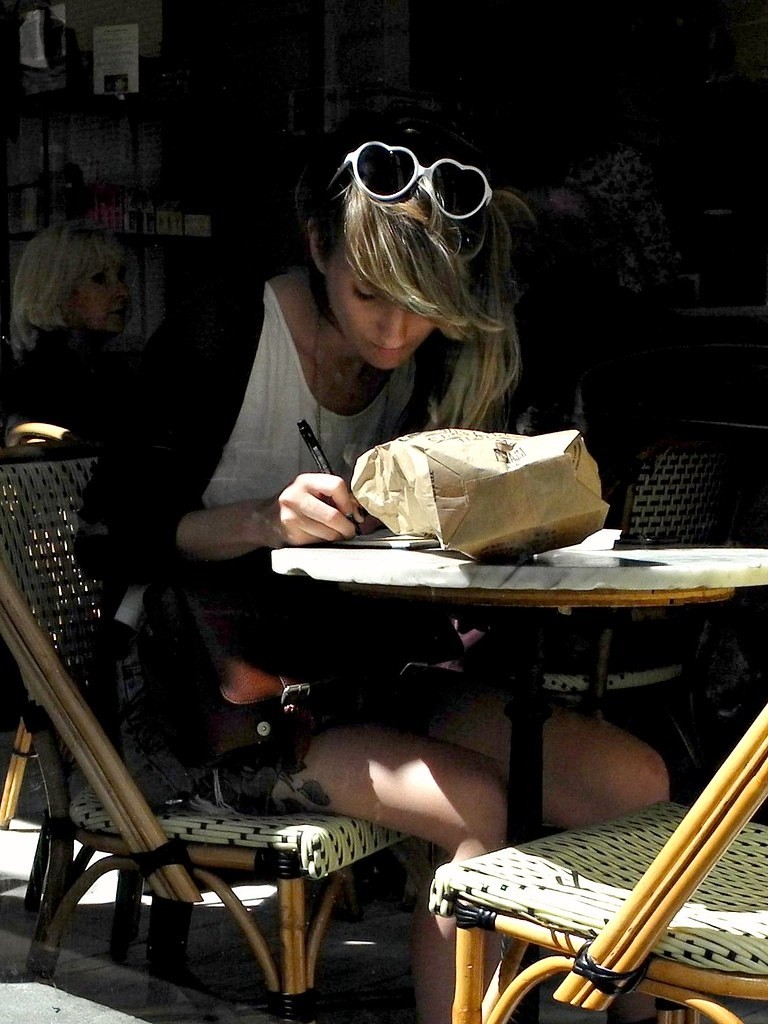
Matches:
[325,528,440,549]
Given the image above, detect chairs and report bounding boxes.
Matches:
[530,415,768,777]
[427,701,768,1024]
[0,438,419,1021]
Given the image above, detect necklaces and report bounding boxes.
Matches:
[313,311,390,455]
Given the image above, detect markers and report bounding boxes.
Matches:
[297,419,363,536]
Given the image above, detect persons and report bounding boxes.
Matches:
[8,219,135,429]
[105,110,669,1024]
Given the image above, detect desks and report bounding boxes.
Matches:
[273,541,768,1024]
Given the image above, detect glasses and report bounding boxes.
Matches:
[323,141,493,220]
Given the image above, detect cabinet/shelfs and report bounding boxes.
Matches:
[0,84,151,345]
